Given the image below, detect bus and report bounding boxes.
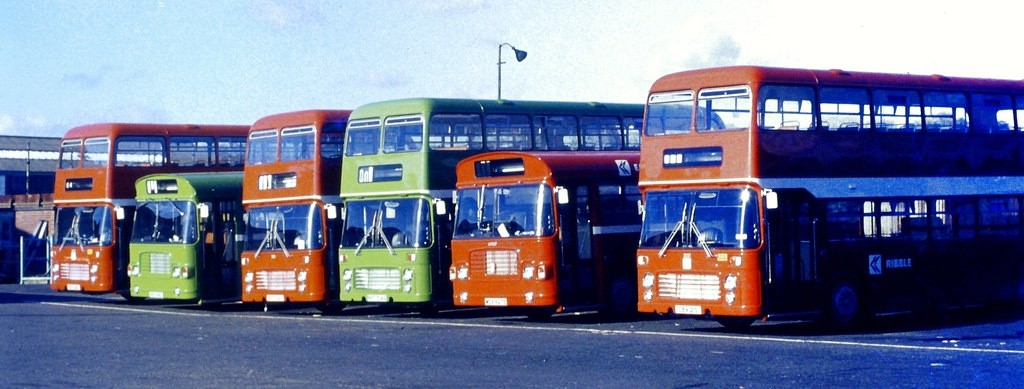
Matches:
[637,65,1024,335]
[339,98,645,317]
[240,109,353,315]
[449,151,641,323]
[50,122,253,305]
[127,171,244,311]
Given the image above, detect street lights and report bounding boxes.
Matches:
[496,42,528,99]
